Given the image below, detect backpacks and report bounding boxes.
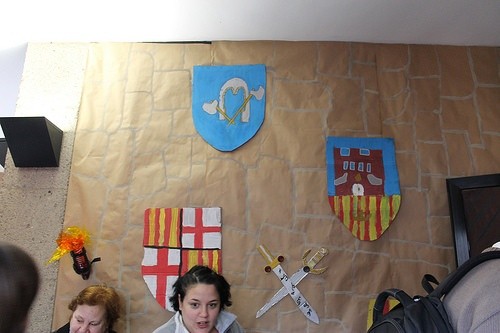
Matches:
[366,249,500,333]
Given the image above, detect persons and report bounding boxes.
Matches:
[152,265,244,333]
[53,285,121,333]
[0,244,39,333]
[442,242,500,333]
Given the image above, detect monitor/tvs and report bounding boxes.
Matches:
[446,173,500,268]
